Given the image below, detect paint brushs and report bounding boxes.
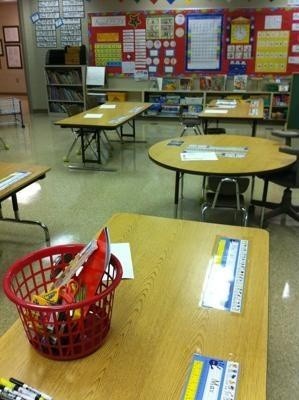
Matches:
[9,378,52,400]
[0,385,33,400]
[0,378,44,400]
[1,390,21,400]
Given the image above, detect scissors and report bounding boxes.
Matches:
[58,279,79,317]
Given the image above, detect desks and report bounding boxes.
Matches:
[1,212,270,400]
[53,99,298,227]
[1,162,52,243]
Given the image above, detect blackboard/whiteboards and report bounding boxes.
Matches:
[285,72,299,132]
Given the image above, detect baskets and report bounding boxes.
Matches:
[3,241,127,362]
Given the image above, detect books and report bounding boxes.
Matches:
[51,226,112,354]
[199,74,227,91]
[50,103,80,112]
[47,68,81,86]
[48,87,84,102]
[233,74,248,93]
[217,99,237,108]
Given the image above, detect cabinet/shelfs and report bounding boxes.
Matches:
[86,72,299,128]
[43,65,86,117]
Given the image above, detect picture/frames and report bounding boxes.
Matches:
[0,38,4,56]
[3,26,20,42]
[5,44,24,69]
